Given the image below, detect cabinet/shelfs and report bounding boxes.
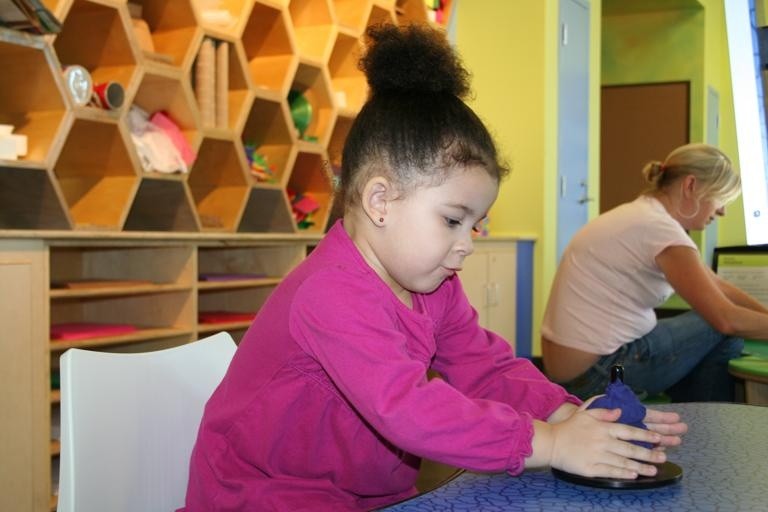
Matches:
[0,228,329,511]
[0,0,437,240]
[456,236,536,361]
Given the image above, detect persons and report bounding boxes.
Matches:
[179,20,690,512]
[535,139,767,405]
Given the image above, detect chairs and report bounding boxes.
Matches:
[51,330,238,511]
